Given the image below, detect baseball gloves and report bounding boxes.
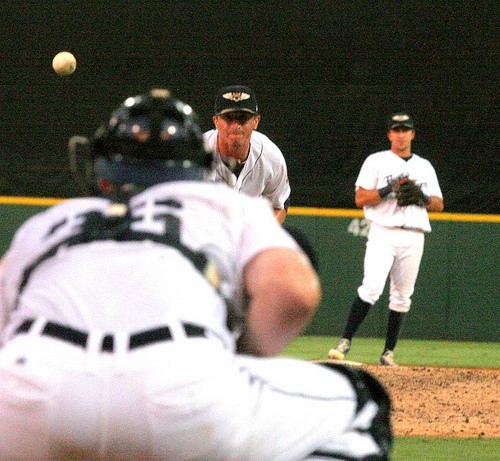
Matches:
[396,179,431,209]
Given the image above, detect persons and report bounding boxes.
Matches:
[0,90,391,461]
[328,113,443,367]
[201,85,290,341]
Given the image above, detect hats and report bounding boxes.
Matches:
[216,85,259,115]
[390,114,413,129]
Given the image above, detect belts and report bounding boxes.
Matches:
[18,321,206,350]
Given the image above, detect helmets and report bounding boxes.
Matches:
[68,92,213,197]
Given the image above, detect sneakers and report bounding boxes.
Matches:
[328,338,351,359]
[381,350,398,366]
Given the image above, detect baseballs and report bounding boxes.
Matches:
[53,50,76,76]
